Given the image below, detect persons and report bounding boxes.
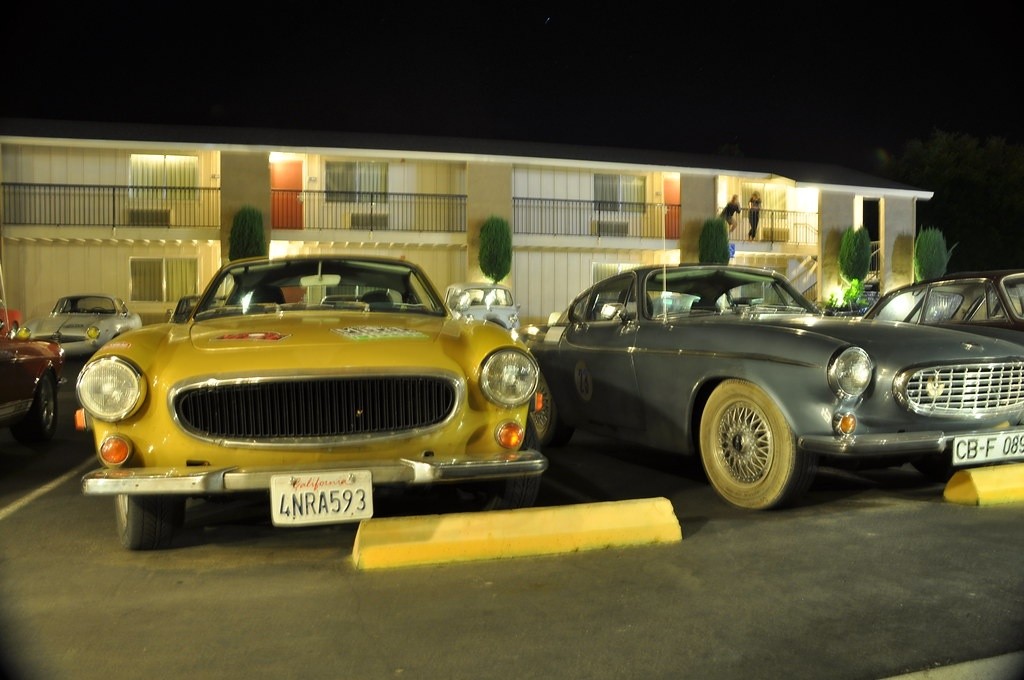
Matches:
[748,191,761,240]
[720,194,741,232]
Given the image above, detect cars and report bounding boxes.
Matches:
[0,298,26,345]
[525,262,1024,511]
[75,252,549,549]
[444,282,522,345]
[8,291,144,367]
[0,332,69,457]
[859,269,1024,336]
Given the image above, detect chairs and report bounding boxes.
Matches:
[361,290,394,313]
[690,300,722,313]
[239,284,287,306]
[617,289,653,319]
